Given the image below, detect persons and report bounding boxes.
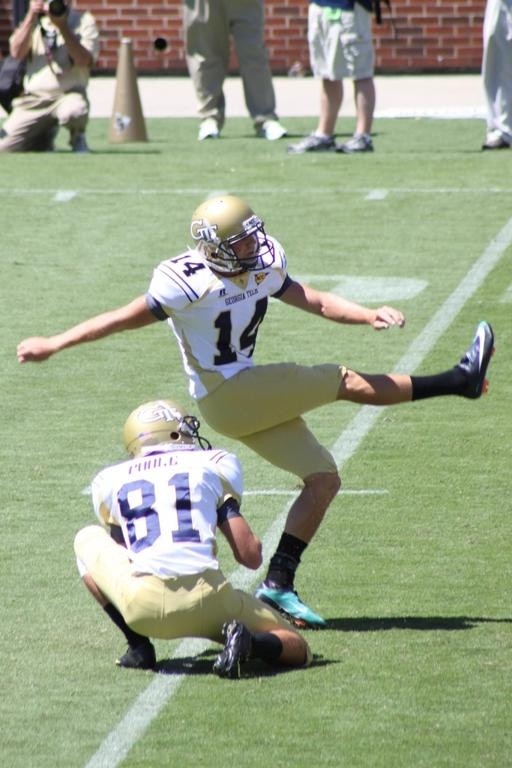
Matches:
[478,1,512,150]
[182,1,289,140]
[1,2,99,153]
[75,398,314,678]
[17,195,495,628]
[286,0,377,154]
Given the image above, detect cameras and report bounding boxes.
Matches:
[48,0,69,17]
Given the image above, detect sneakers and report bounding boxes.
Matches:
[254,581,328,629]
[454,321,493,398]
[115,644,156,669]
[481,135,509,149]
[212,619,255,678]
[197,117,374,153]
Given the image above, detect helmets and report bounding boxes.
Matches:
[190,196,274,273]
[122,400,200,459]
